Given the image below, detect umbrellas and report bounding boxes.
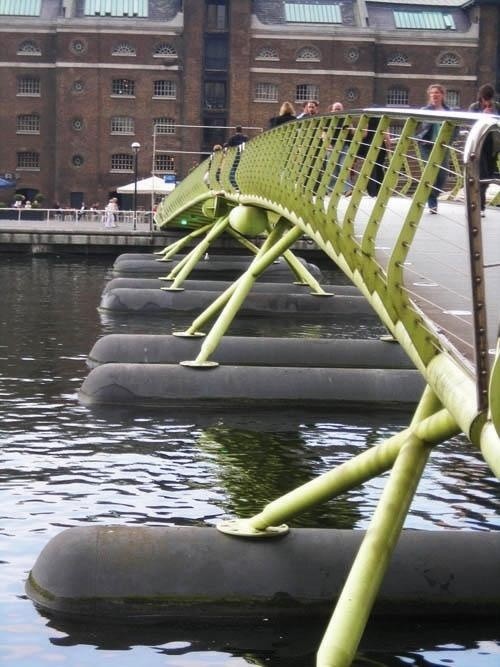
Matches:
[0,177,16,189]
[115,174,177,210]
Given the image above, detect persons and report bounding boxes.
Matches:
[268,100,296,128]
[12,196,120,227]
[467,80,500,216]
[360,102,393,197]
[415,82,459,216]
[227,124,249,190]
[295,100,308,119]
[322,101,357,196]
[188,160,198,173]
[210,144,226,181]
[301,100,327,195]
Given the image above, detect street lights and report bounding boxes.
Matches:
[130,140,142,230]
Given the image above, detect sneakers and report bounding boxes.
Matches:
[429,206,437,215]
